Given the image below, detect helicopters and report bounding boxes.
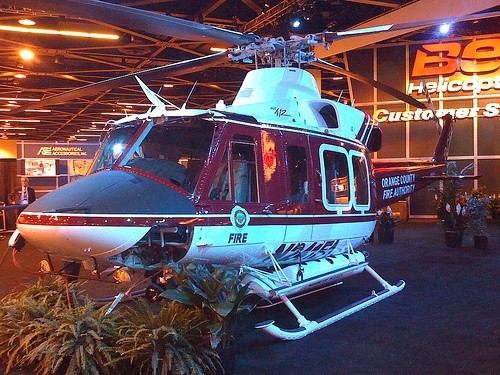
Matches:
[0,0,483,343]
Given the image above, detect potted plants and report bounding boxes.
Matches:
[429,166,489,249]
[378,211,396,242]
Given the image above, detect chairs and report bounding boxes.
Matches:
[209,160,248,204]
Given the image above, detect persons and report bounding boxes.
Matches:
[9,177,35,220]
